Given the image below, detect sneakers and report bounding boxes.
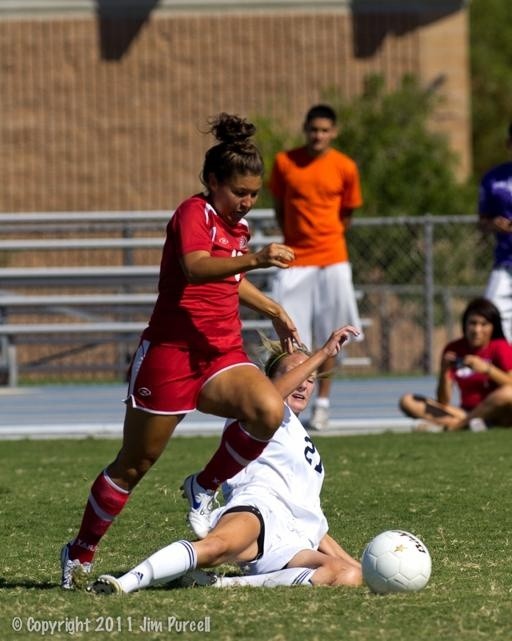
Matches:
[179,472,218,540]
[180,569,214,588]
[92,574,124,598]
[59,542,93,591]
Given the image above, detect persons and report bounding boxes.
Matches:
[58,109,303,590]
[265,103,363,431]
[398,294,512,435]
[476,164,512,345]
[90,322,367,597]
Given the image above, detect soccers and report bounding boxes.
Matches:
[362,529,431,593]
